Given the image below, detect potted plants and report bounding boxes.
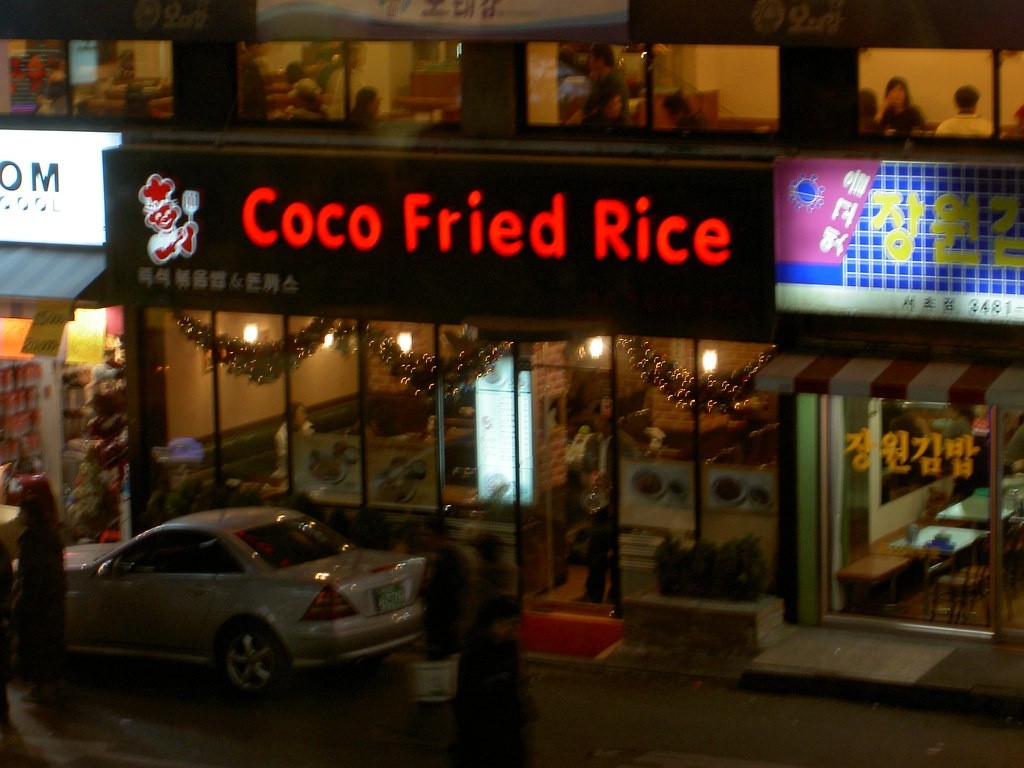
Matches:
[622,535,784,646]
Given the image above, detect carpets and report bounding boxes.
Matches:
[517,610,626,662]
[915,643,1024,699]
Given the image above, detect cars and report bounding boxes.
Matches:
[7,505,427,706]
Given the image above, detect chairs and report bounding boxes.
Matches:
[375,460,429,503]
[955,528,1013,620]
[927,535,989,623]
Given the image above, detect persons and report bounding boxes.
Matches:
[285,494,538,768]
[10,40,993,137]
[940,404,971,476]
[569,402,642,620]
[11,499,66,703]
[1002,413,1024,477]
[880,402,934,505]
[0,539,11,728]
[950,416,991,571]
[275,400,314,475]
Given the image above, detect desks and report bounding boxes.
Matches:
[936,493,1024,563]
[835,553,915,615]
[889,524,988,613]
[977,473,1024,534]
